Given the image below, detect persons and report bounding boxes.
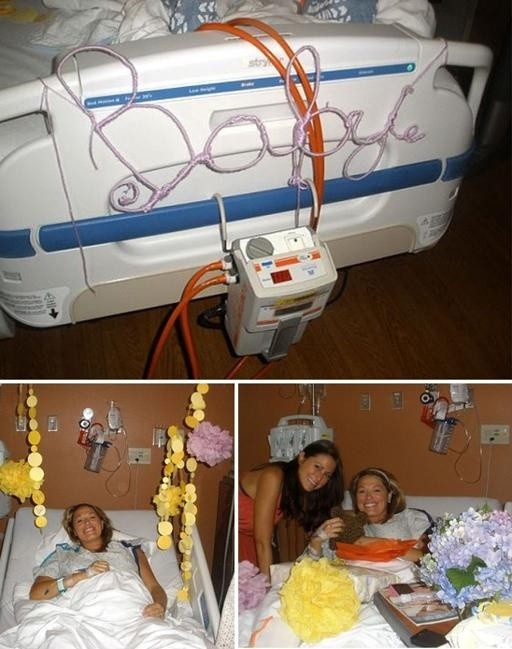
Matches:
[407,506,435,549]
[239,437,346,584]
[31,500,168,620]
[249,466,437,646]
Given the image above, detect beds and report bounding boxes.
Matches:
[0,0,493,337]
[239,491,502,647]
[0,508,221,649]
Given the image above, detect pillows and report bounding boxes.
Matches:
[35,521,156,569]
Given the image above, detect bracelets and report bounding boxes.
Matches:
[311,527,330,541]
[56,576,67,593]
[83,563,100,577]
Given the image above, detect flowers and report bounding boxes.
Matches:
[415,502,512,620]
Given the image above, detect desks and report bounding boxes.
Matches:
[373,580,460,648]
